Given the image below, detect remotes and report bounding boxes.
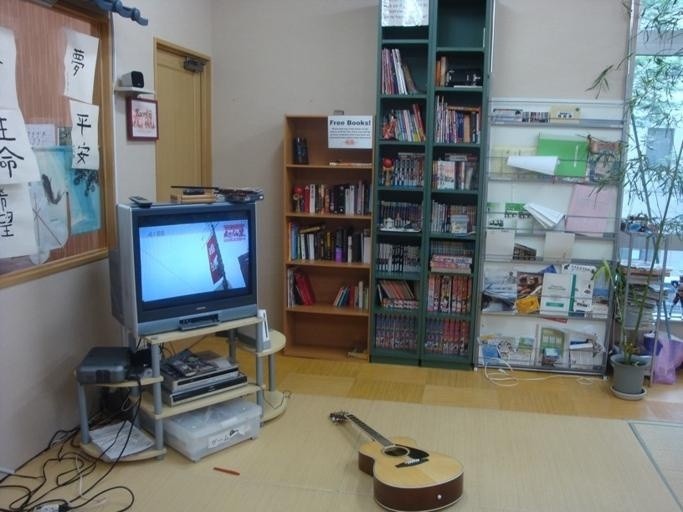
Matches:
[128,195,152,208]
[169,359,196,377]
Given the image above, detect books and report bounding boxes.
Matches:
[481,104,625,374]
[346,343,370,360]
[619,255,671,338]
[373,43,482,358]
[286,159,373,311]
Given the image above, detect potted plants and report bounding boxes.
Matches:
[578,0,683,400]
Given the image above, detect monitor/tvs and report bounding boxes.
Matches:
[109,200,258,338]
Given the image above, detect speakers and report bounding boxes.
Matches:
[121,71,144,88]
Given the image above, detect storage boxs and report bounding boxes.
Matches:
[139,398,262,463]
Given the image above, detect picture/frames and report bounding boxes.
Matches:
[126,96,159,141]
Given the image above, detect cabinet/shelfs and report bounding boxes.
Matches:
[283,114,374,363]
[73,316,287,463]
[369,0,494,372]
[610,231,671,388]
[473,97,628,379]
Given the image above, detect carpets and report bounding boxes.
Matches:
[0,393,682,512]
[628,421,683,512]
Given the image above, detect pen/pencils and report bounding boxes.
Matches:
[213,467,238,476]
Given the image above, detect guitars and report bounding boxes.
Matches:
[330,410,464,512]
